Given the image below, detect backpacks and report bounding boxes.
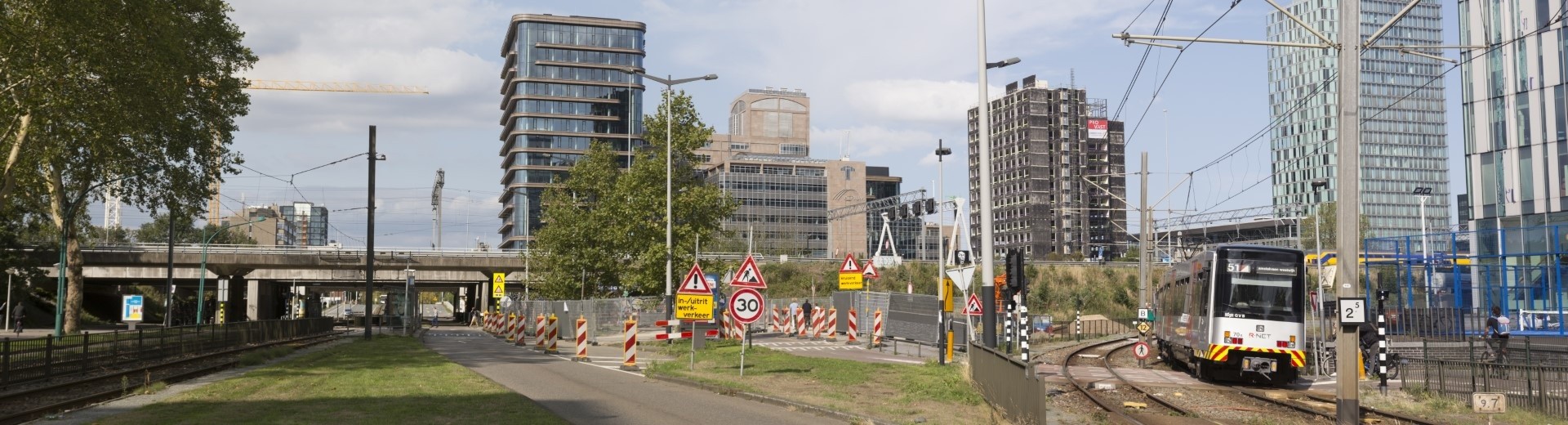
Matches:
[1491,314,1510,335]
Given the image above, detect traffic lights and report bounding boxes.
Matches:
[1006,250,1021,295]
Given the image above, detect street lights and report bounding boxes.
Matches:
[616,65,718,340]
[935,138,952,347]
[1311,178,1328,374]
[978,0,1021,346]
[4,268,16,331]
[197,215,266,326]
[1411,186,1432,331]
[53,163,164,340]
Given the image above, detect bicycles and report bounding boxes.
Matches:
[1479,334,1511,380]
[1321,332,1399,380]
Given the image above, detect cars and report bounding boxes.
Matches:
[1524,312,1561,331]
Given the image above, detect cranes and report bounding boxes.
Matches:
[178,55,430,226]
[431,167,445,250]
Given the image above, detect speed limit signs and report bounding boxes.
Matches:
[729,288,765,324]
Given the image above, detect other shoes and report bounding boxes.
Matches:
[1366,373,1374,379]
[1486,356,1495,361]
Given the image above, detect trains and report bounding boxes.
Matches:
[1155,242,1312,387]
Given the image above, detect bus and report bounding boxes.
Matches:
[379,295,388,304]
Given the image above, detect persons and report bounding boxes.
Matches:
[1330,320,1379,376]
[815,304,821,313]
[11,302,26,333]
[469,307,479,328]
[802,300,812,327]
[431,307,438,328]
[1481,306,1508,362]
[789,297,799,330]
